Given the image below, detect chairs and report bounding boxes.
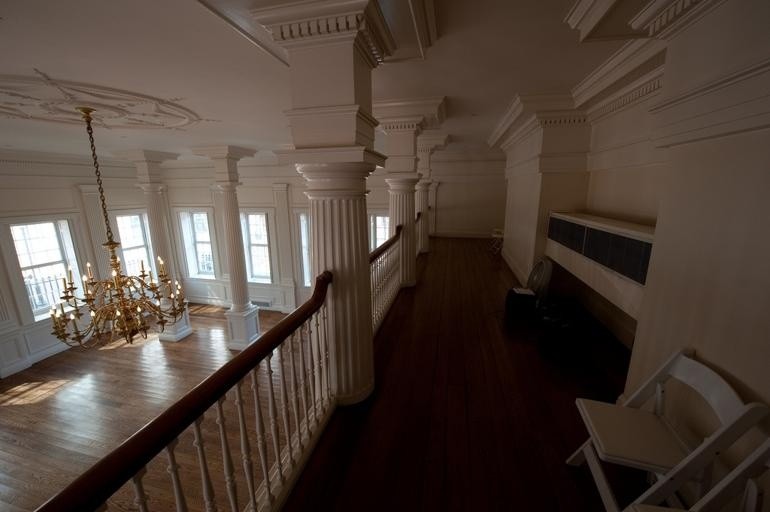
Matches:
[562,342,769,512]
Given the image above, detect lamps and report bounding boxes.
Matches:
[49,106,190,353]
